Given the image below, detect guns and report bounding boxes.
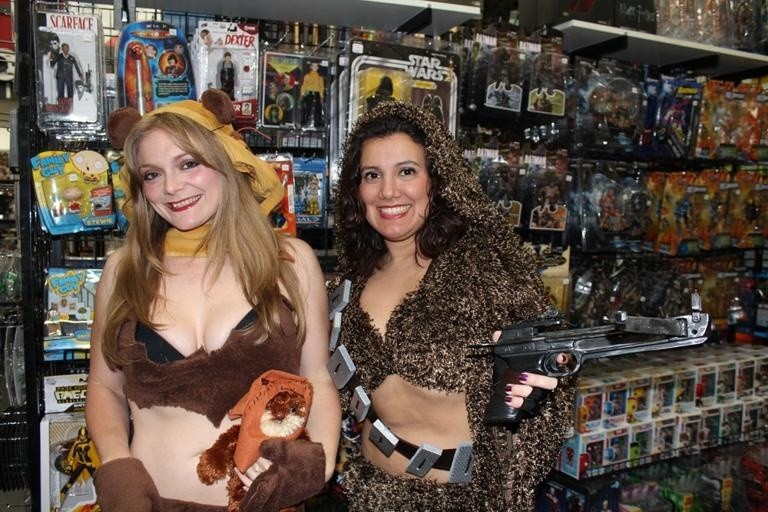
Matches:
[466,311,709,427]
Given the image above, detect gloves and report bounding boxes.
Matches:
[93,457,161,512]
[238,437,327,512]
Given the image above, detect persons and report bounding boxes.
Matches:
[328,101,576,512]
[83,89,343,512]
[300,61,326,129]
[51,43,84,99]
[219,52,238,101]
